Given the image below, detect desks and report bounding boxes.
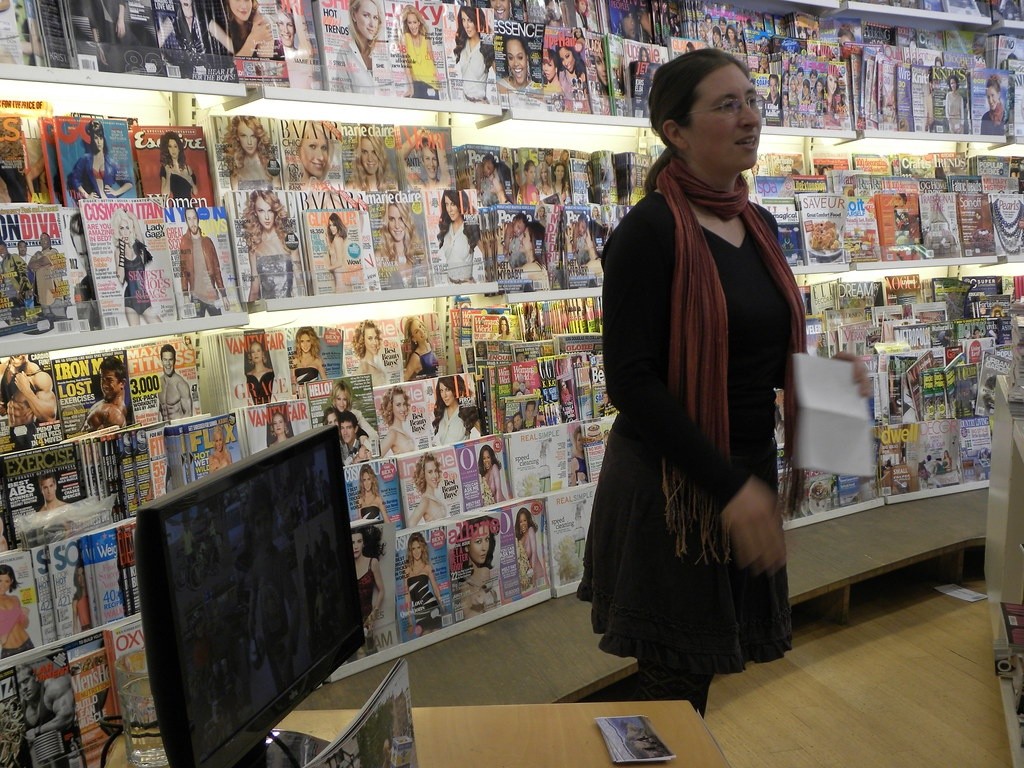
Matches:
[104,697,732,768]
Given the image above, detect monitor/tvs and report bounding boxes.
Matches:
[134,423,367,768]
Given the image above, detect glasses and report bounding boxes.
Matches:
[686,94,766,117]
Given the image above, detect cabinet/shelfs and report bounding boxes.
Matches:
[0,0,1024,767]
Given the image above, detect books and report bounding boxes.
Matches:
[0,0,1024,768]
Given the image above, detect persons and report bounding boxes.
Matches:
[578,50,876,719]
